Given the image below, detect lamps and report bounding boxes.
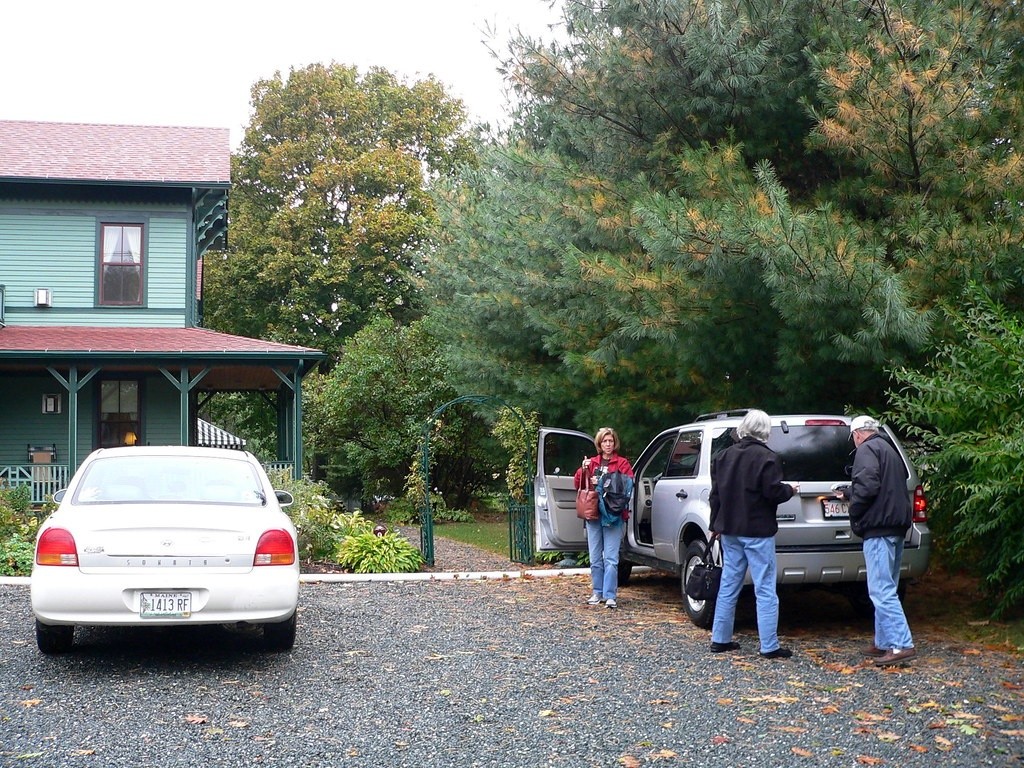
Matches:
[125,432,137,445]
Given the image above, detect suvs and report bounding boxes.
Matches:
[535,406,933,627]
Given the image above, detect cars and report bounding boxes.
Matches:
[31,445,300,653]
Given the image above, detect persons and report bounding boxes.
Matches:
[710,410,794,659]
[834,415,919,666]
[575,427,634,608]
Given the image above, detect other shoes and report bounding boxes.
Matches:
[587,595,600,604]
[710,642,740,652]
[874,648,917,665]
[606,599,617,607]
[860,642,886,657]
[760,648,792,659]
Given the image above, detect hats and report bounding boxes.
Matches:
[848,416,876,441]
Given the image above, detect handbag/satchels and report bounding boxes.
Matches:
[685,532,724,600]
[576,467,599,520]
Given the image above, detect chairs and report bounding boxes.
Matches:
[28,443,69,490]
